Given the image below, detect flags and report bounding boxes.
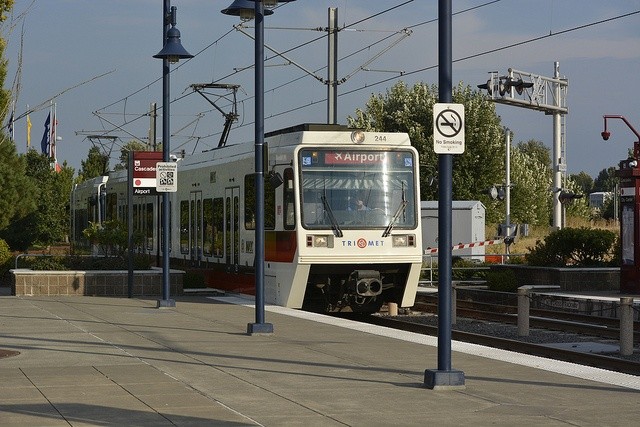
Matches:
[50,113,57,162]
[55,163,61,173]
[8,112,13,144]
[40,111,50,157]
[27,115,32,145]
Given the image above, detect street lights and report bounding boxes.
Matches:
[220,1,274,333]
[154,7,195,306]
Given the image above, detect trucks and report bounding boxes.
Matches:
[422,200,486,274]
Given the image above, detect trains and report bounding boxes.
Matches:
[69,124,423,314]
